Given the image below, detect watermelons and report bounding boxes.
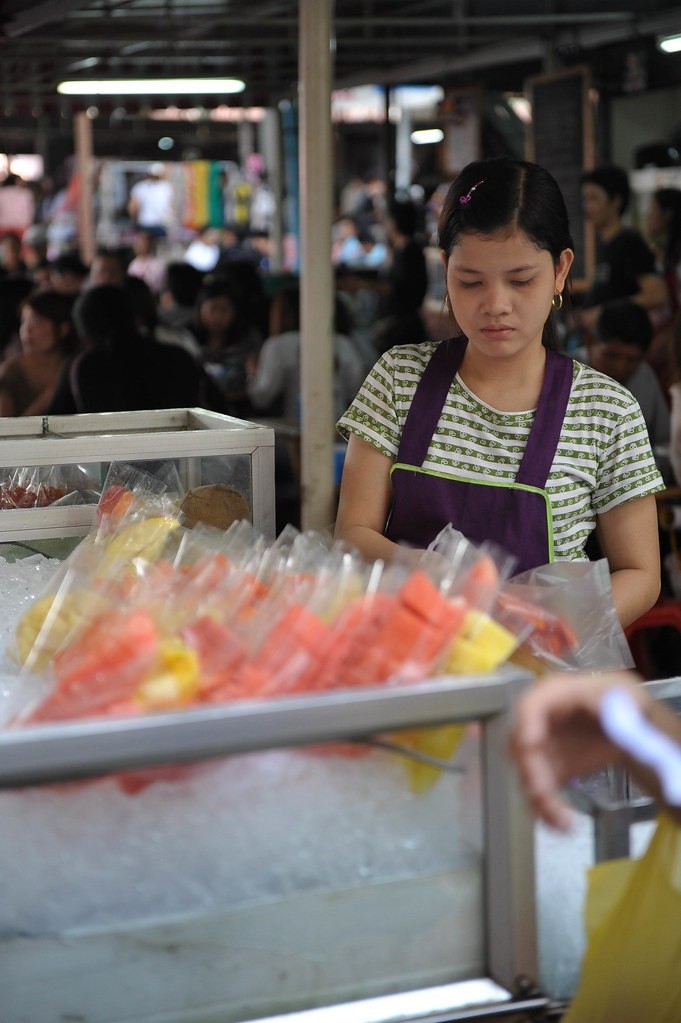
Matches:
[11,554,467,792]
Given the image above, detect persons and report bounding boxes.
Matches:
[331,154,667,633]
[507,669,681,842]
[0,152,681,528]
